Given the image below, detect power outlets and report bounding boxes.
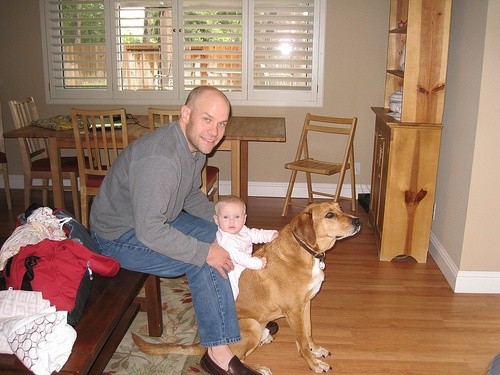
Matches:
[353,161,361,176]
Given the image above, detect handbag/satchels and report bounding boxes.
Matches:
[4,214,121,324]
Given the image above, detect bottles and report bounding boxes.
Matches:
[388,89,402,113]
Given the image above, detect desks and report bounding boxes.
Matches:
[0,267,164,375]
[2,115,287,206]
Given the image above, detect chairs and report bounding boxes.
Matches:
[71,107,129,231]
[281,113,358,217]
[147,107,221,204]
[7,97,95,223]
[0,102,13,211]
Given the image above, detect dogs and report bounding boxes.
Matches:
[130,200,362,375]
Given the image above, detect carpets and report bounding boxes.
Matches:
[99,273,199,375]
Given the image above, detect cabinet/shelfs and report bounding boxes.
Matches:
[366,106,443,264]
[384,0,454,124]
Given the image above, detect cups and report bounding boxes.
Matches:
[398,22,407,28]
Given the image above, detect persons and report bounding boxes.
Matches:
[89,85,262,375]
[213,195,279,301]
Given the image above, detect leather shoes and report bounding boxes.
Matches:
[265,321,279,335]
[200,351,255,375]
